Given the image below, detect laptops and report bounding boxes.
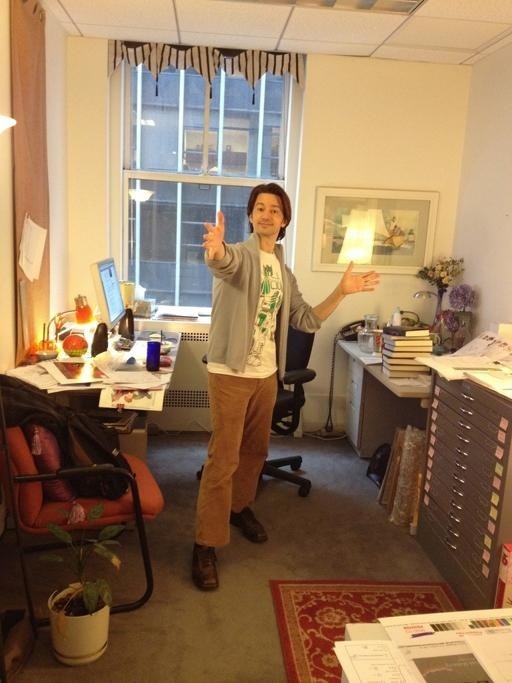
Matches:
[85,411,139,433]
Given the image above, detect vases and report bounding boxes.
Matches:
[433,292,443,326]
[45,578,112,665]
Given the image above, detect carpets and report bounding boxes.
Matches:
[264,574,462,681]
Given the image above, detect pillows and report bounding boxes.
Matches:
[27,424,74,501]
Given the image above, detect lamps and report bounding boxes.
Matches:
[34,291,95,360]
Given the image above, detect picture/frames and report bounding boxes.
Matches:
[308,183,441,277]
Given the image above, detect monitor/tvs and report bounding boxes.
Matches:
[90,257,126,342]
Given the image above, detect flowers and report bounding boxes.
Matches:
[41,500,139,606]
[448,282,478,312]
[439,308,461,347]
[414,254,465,313]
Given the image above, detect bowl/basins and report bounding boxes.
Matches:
[36,350,60,360]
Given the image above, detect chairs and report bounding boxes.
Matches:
[0,367,172,638]
[193,324,317,500]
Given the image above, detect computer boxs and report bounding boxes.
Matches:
[117,416,149,470]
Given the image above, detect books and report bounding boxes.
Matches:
[463,370,511,391]
[156,305,199,320]
[381,324,434,379]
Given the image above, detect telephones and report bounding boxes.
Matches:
[340,320,365,341]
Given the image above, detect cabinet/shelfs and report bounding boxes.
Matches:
[417,364,512,613]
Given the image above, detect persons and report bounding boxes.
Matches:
[190,183,382,589]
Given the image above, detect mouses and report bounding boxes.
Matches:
[147,332,166,339]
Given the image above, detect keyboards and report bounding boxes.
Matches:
[115,340,149,372]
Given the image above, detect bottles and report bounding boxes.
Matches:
[392,306,401,327]
[357,313,379,352]
[120,281,133,311]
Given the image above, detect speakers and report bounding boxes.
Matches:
[91,323,109,357]
[118,309,135,342]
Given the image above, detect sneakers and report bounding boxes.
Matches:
[192,542,219,590]
[229,505,268,544]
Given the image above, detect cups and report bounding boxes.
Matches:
[146,340,162,371]
[374,330,383,352]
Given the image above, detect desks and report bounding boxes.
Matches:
[14,327,182,465]
[329,337,435,461]
